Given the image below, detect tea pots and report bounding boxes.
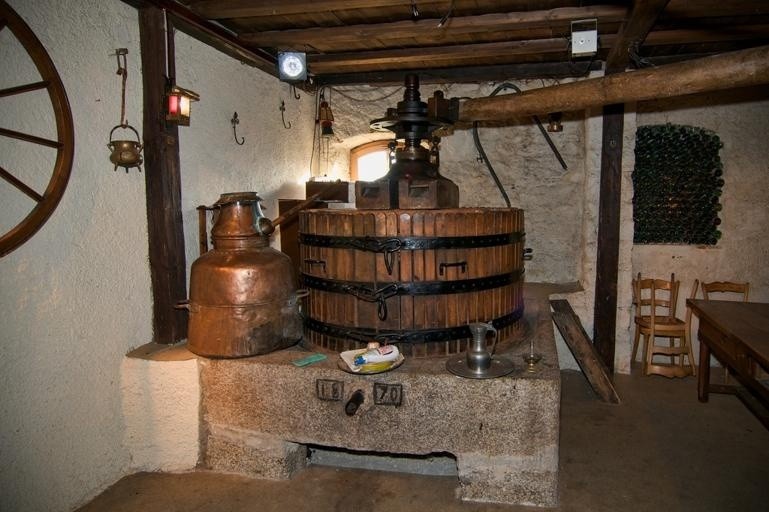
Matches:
[465,321,500,372]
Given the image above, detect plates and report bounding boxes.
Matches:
[446,353,516,380]
[339,348,406,376]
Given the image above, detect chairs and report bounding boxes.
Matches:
[631,271,751,382]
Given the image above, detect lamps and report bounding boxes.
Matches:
[168,83,197,125]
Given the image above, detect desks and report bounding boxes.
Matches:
[684,299,768,429]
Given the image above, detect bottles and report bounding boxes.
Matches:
[352,344,399,365]
